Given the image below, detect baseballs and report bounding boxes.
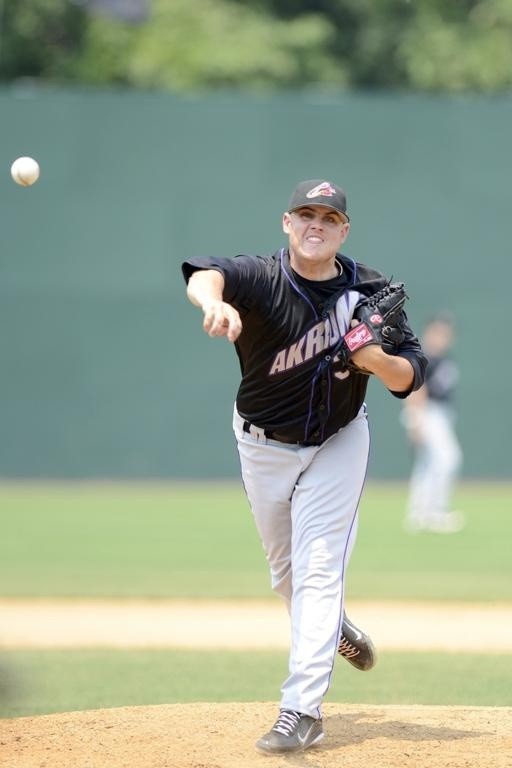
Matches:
[10,156,39,186]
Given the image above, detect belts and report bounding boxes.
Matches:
[243,421,307,444]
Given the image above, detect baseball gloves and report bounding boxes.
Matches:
[339,275,409,376]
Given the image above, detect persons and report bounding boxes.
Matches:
[403,316,464,533]
[181,180,427,756]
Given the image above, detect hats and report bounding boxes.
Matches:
[288,179,350,222]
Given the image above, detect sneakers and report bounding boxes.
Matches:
[254,709,324,755]
[337,610,376,672]
